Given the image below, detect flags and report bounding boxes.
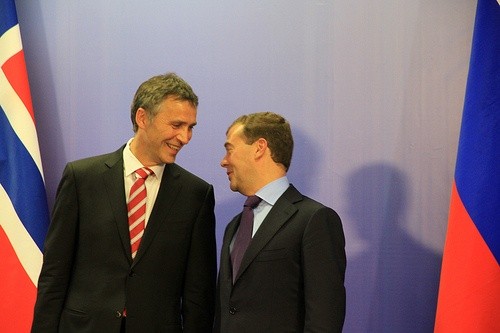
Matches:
[215,112,346,333]
[434,0,500,333]
[0,1,49,333]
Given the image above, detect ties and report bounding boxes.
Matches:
[230,195,262,285]
[127,167,154,259]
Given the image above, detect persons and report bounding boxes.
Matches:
[30,73,217,333]
